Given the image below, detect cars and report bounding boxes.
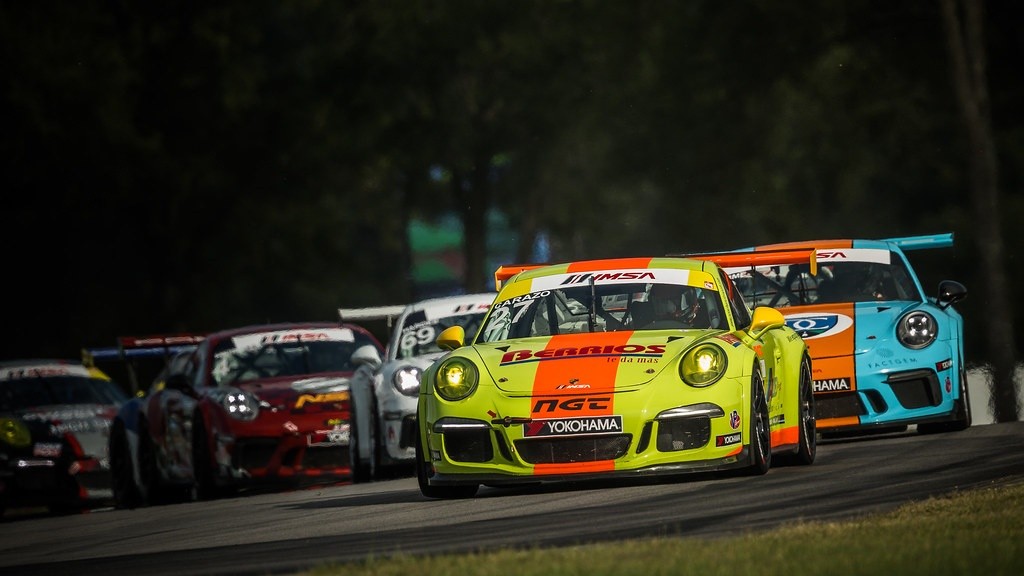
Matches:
[664,231,973,444]
[337,291,501,484]
[0,359,129,517]
[416,247,818,501]
[87,322,385,510]
